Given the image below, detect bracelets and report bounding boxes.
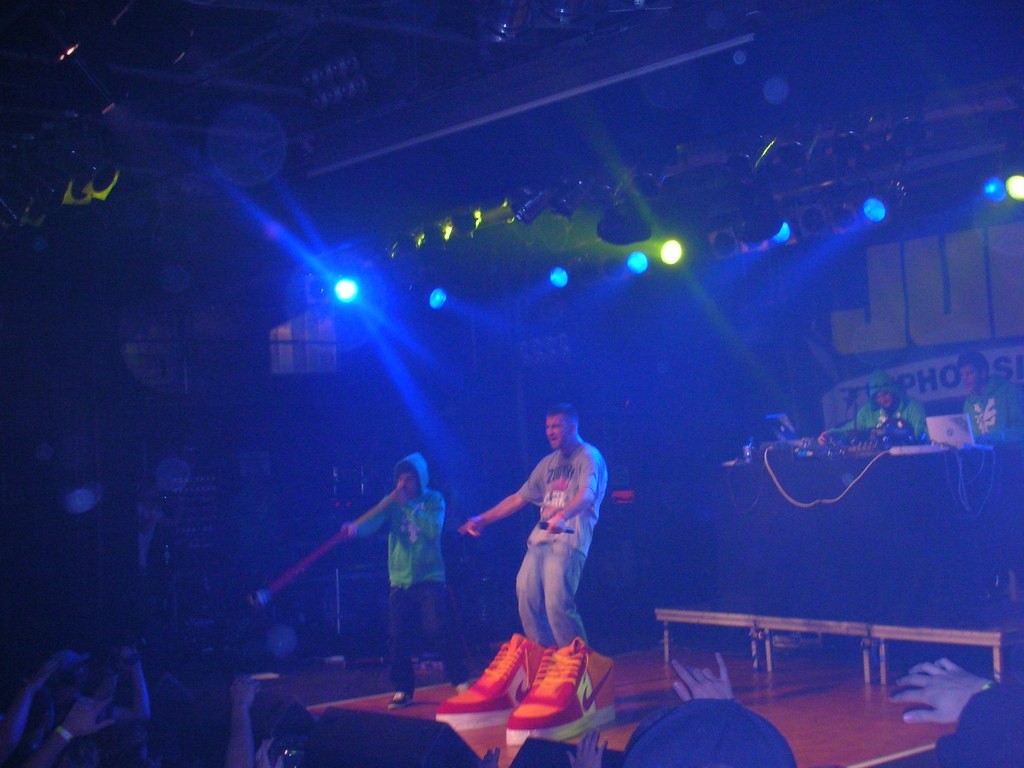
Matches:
[55,726,74,743]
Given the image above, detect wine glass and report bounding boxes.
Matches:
[282,737,304,768]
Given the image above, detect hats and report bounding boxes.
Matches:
[620,698,797,768]
[937,684,1024,768]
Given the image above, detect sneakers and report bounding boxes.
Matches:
[434,633,544,732]
[505,636,617,747]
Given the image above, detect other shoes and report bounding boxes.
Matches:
[388,691,413,709]
[456,680,470,694]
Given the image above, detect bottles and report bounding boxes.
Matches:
[877,408,888,443]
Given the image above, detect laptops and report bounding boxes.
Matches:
[767,414,801,442]
[927,413,993,450]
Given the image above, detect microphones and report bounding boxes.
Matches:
[539,521,575,534]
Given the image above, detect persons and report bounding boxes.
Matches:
[347,455,472,709]
[458,404,607,648]
[817,368,926,448]
[957,351,1024,445]
[0,649,1024,768]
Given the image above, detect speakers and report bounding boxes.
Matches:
[245,684,628,767]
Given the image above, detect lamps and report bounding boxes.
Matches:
[366,102,925,289]
